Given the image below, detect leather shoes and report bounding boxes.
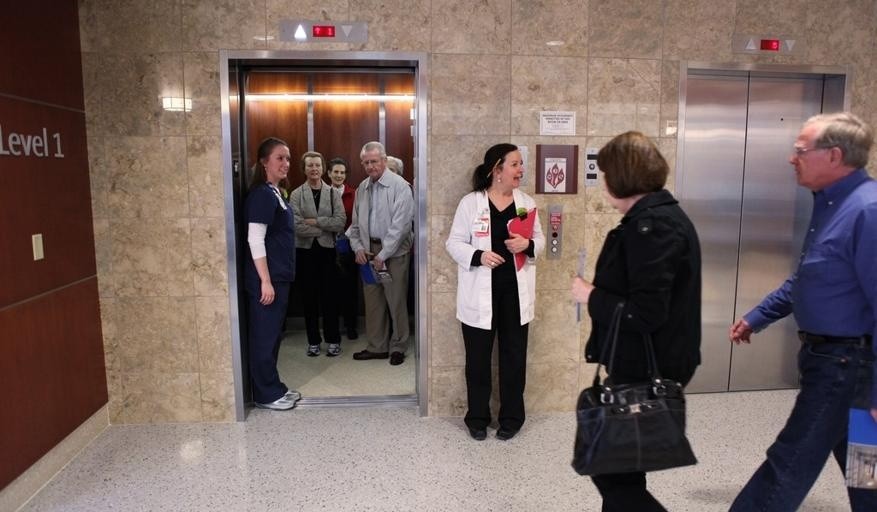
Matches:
[496,425,516,440]
[390,351,404,364]
[470,425,486,440]
[353,350,389,360]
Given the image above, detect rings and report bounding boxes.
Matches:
[490,262,494,266]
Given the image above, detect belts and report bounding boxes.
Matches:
[371,238,381,245]
[798,330,871,346]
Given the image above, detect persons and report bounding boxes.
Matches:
[443,143,546,442]
[727,110,876,511]
[248,141,415,365]
[570,129,703,511]
[239,136,302,410]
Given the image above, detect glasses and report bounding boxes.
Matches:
[794,144,837,153]
[361,159,379,165]
[500,161,523,168]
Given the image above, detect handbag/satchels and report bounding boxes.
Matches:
[335,232,349,270]
[571,377,697,474]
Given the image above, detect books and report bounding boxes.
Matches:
[505,207,537,271]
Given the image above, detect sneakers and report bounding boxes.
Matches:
[327,344,343,356]
[284,390,301,401]
[347,328,358,340]
[254,396,296,410]
[307,345,320,356]
